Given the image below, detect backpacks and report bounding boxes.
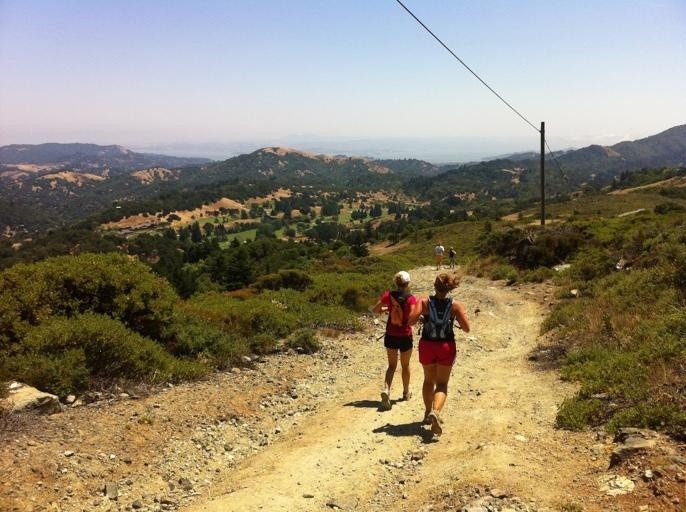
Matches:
[389,292,404,327]
[424,295,454,341]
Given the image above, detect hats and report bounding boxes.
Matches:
[394,270,410,284]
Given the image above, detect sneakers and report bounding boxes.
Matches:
[428,411,443,434]
[422,411,432,424]
[403,389,412,401]
[379,389,392,410]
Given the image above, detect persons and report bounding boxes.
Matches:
[434,242,445,271]
[371,270,419,410]
[447,246,457,269]
[408,273,471,435]
